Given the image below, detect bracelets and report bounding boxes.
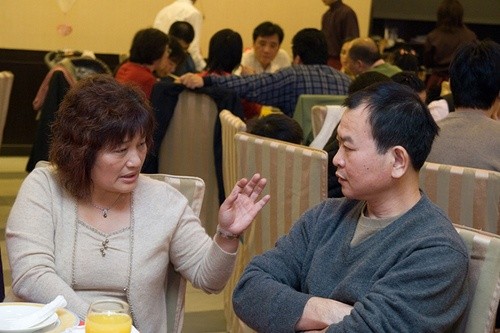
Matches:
[217,224,239,240]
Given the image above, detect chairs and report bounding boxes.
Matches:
[0,73,500,333]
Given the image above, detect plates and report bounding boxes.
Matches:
[0,301,80,333]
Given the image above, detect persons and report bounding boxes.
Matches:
[232,80,476,333]
[154,0,208,73]
[4,76,271,333]
[198,29,261,121]
[426,41,500,172]
[180,27,352,118]
[168,21,196,76]
[115,27,170,105]
[426,93,453,123]
[252,114,304,144]
[424,0,479,70]
[339,37,359,80]
[391,71,426,102]
[346,36,402,77]
[233,21,291,76]
[321,0,359,72]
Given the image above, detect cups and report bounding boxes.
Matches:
[385,27,398,47]
[85,299,132,333]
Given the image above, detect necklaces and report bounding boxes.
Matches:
[93,203,110,217]
